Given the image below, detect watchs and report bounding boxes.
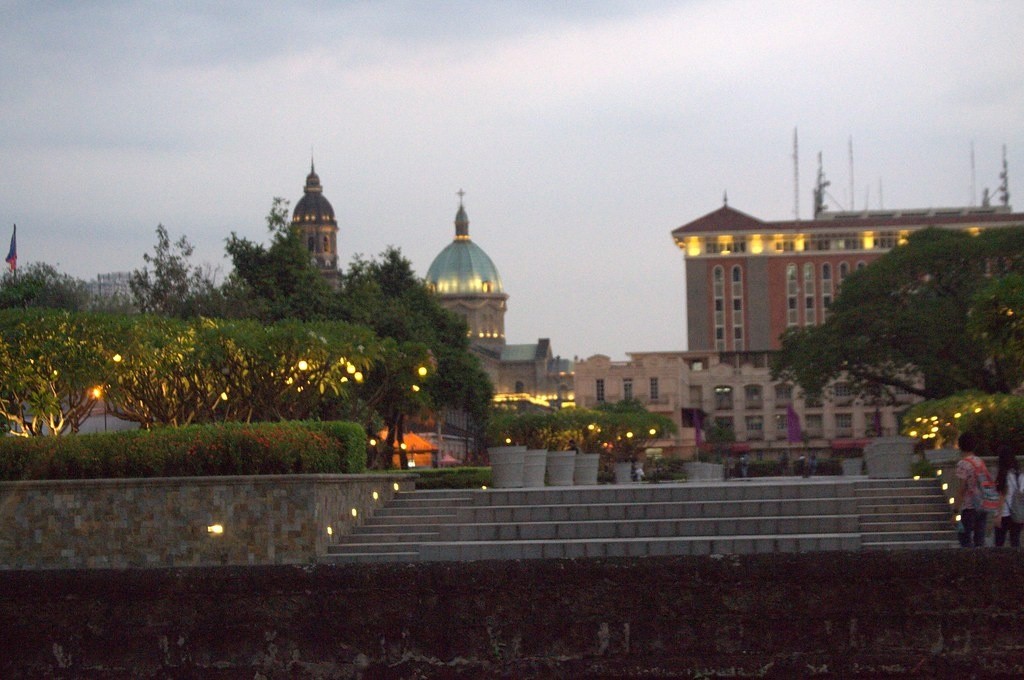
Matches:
[952,507,959,514]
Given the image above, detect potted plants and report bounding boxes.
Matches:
[546,408,585,487]
[602,412,679,484]
[518,408,557,486]
[839,446,863,476]
[574,410,600,487]
[482,412,527,487]
[685,416,736,481]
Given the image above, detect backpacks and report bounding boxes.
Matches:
[963,458,1001,513]
[1004,471,1024,524]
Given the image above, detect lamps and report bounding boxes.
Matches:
[93,383,112,398]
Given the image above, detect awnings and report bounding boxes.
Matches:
[701,441,750,452]
[830,438,870,449]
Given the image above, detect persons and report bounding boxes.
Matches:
[993,446,1024,549]
[717,450,819,479]
[949,431,996,550]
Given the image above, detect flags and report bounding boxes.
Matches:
[694,409,704,450]
[4,224,18,273]
[787,404,805,442]
